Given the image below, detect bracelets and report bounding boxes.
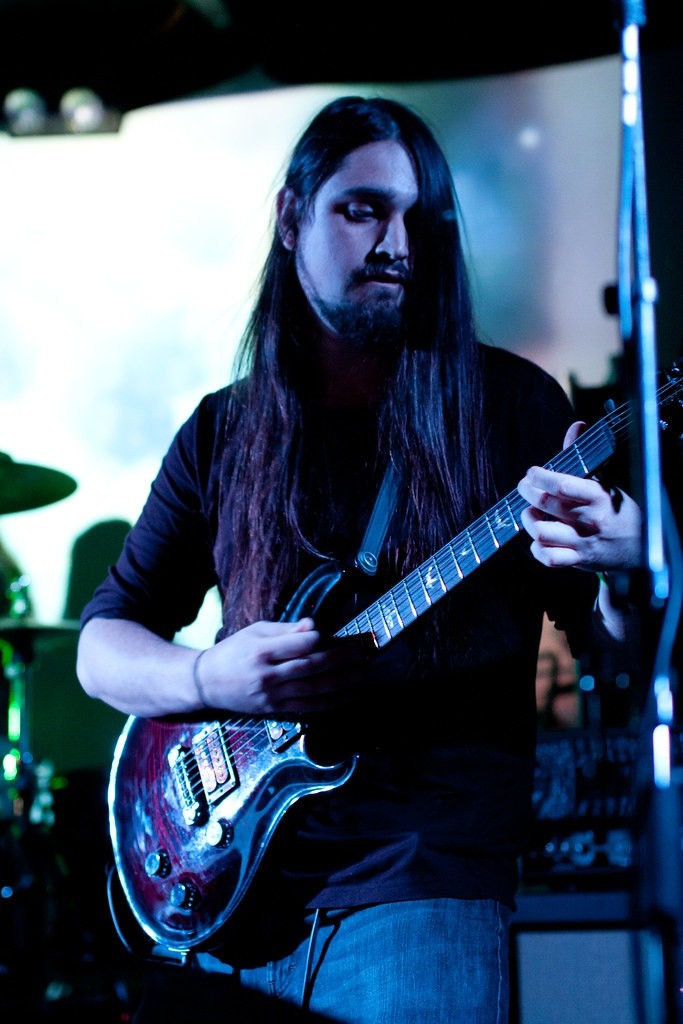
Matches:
[193,647,214,710]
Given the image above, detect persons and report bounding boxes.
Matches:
[75,98,641,1024]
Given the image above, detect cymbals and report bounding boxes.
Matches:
[0,452,78,514]
[0,618,85,643]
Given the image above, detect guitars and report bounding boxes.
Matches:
[108,341,683,963]
